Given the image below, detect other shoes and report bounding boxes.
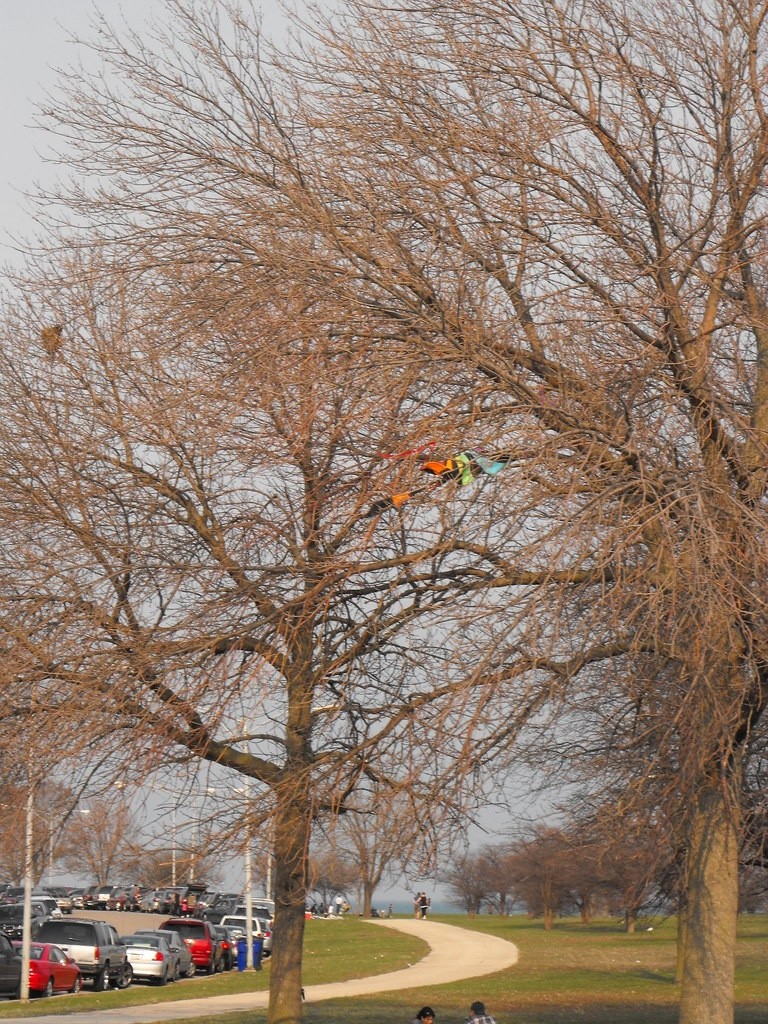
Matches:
[186,915,189,917]
[181,916,184,918]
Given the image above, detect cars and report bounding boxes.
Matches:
[0,930,23,1000]
[11,941,82,997]
[192,891,276,971]
[119,935,181,985]
[627,901,674,918]
[739,904,766,914]
[0,882,85,937]
[82,883,210,917]
[134,930,196,981]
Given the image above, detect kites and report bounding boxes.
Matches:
[357,442,506,518]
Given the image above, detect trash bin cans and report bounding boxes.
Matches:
[238,935,265,973]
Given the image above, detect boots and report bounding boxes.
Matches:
[422,915,426,920]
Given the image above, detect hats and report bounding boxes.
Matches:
[183,899,187,902]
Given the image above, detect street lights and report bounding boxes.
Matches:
[117,781,215,887]
[1,803,91,885]
[189,789,245,883]
[189,704,341,971]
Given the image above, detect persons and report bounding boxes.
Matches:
[173,892,189,918]
[369,904,393,918]
[304,895,347,915]
[463,1001,497,1024]
[413,892,432,920]
[413,1006,436,1024]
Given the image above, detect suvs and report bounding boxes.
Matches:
[158,917,225,975]
[34,917,133,991]
